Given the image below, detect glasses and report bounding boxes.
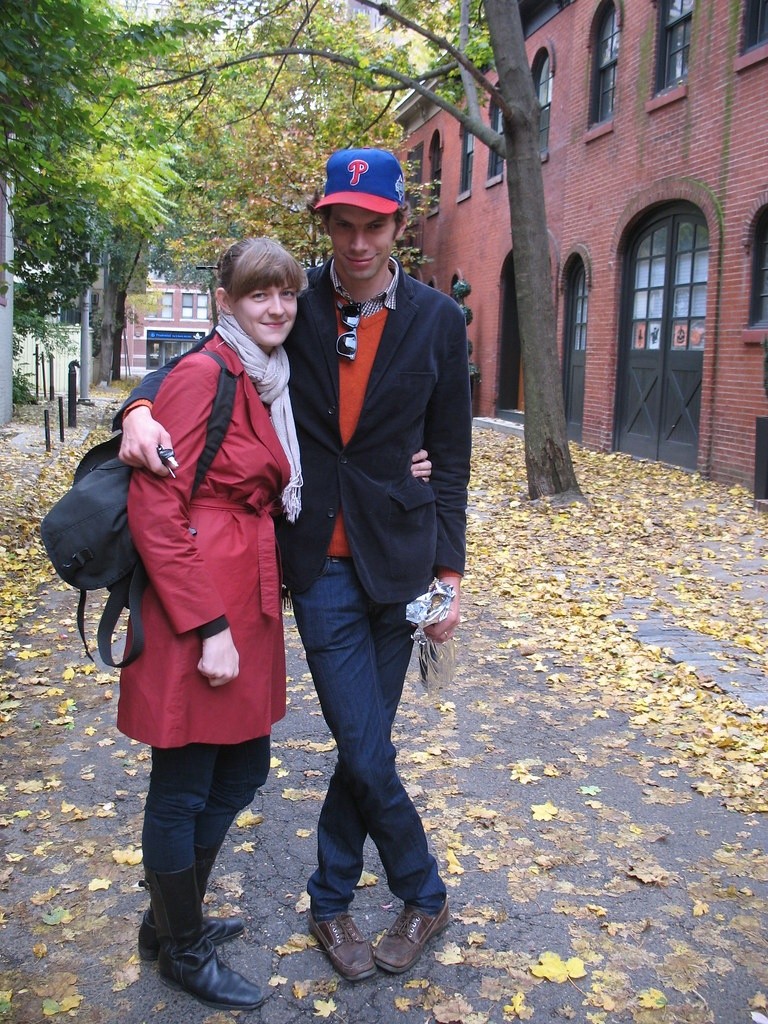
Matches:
[336,302,361,360]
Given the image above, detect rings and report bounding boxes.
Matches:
[444,630,448,638]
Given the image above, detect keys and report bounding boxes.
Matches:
[158,445,179,478]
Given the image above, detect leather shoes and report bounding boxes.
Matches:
[138,861,244,960]
[139,863,265,1010]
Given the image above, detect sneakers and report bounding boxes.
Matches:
[308,909,377,980]
[374,894,450,973]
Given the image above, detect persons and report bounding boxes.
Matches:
[112,150,472,981]
[116,236,433,1010]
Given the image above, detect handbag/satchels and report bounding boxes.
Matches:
[40,457,148,667]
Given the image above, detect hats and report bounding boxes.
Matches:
[315,147,405,214]
